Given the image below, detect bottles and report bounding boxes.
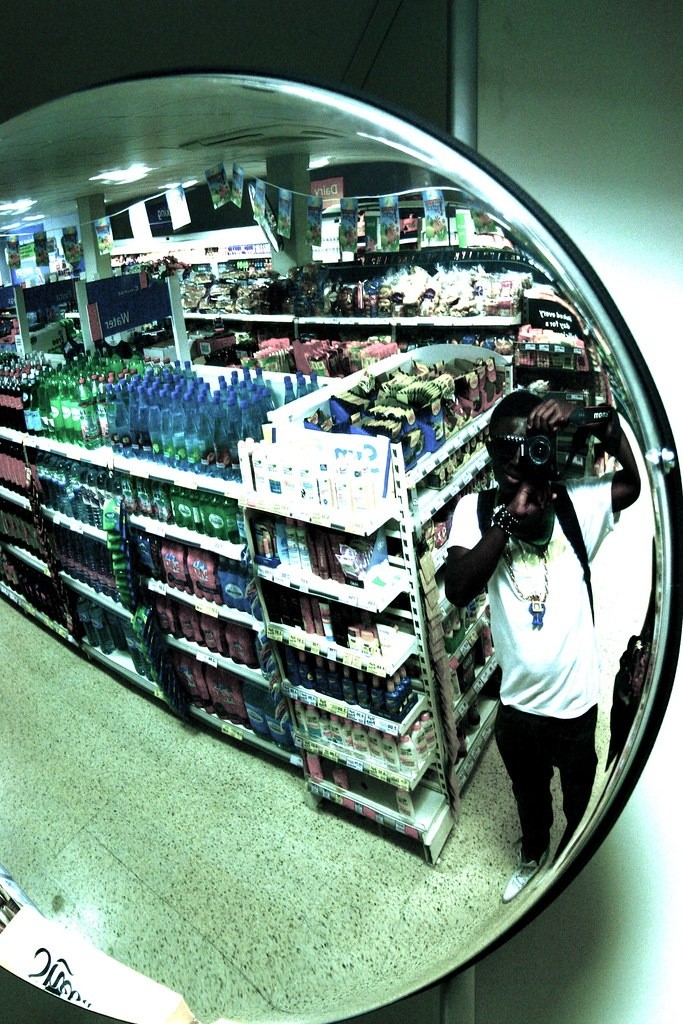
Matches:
[0,320,509,828]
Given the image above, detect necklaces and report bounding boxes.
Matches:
[501,537,550,628]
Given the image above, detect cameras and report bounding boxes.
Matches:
[523,423,556,481]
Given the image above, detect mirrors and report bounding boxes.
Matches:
[0,60,683,1024]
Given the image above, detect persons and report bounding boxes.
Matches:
[440,385,642,910]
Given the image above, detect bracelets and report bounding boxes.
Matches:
[492,507,529,539]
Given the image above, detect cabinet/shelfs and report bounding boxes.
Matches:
[0,249,614,869]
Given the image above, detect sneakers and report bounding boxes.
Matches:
[503,852,547,903]
